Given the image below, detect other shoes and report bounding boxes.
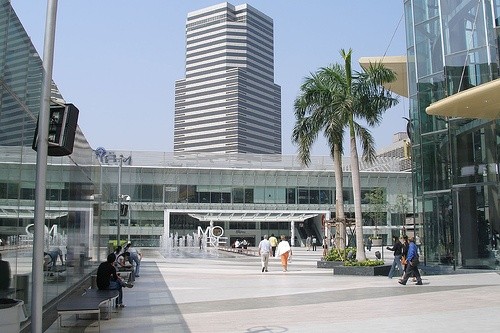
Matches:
[262,268,264,272]
[265,270,268,272]
[135,275,139,277]
[126,284,133,288]
[416,283,422,285]
[399,281,405,285]
[120,304,126,307]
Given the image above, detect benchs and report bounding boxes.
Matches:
[57,271,132,333]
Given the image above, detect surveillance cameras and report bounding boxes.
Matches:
[126,196,131,202]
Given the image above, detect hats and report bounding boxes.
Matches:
[408,236,414,242]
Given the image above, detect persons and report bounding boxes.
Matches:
[415,233,422,255]
[368,235,373,251]
[386,234,406,279]
[96,253,133,307]
[127,247,143,277]
[0,252,11,289]
[323,234,339,256]
[258,235,272,272]
[277,235,291,272]
[234,239,248,248]
[312,236,316,251]
[112,246,122,262]
[398,237,423,285]
[269,233,278,257]
[399,235,417,282]
[116,252,135,281]
[306,236,311,251]
[44,247,64,276]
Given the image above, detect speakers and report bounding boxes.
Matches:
[33,103,79,156]
[120,204,128,216]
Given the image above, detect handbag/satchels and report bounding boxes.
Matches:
[412,256,419,266]
[401,255,407,264]
